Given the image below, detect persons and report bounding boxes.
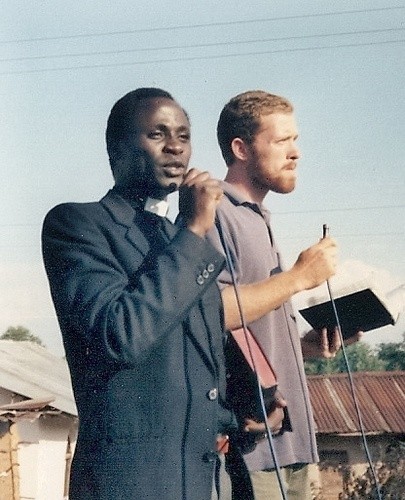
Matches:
[41,87,288,500]
[173,90,364,500]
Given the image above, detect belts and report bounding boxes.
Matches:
[216,435,231,454]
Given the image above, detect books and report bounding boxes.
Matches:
[299,269,404,337]
[225,324,278,456]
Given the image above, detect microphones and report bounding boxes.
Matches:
[322,224,329,239]
